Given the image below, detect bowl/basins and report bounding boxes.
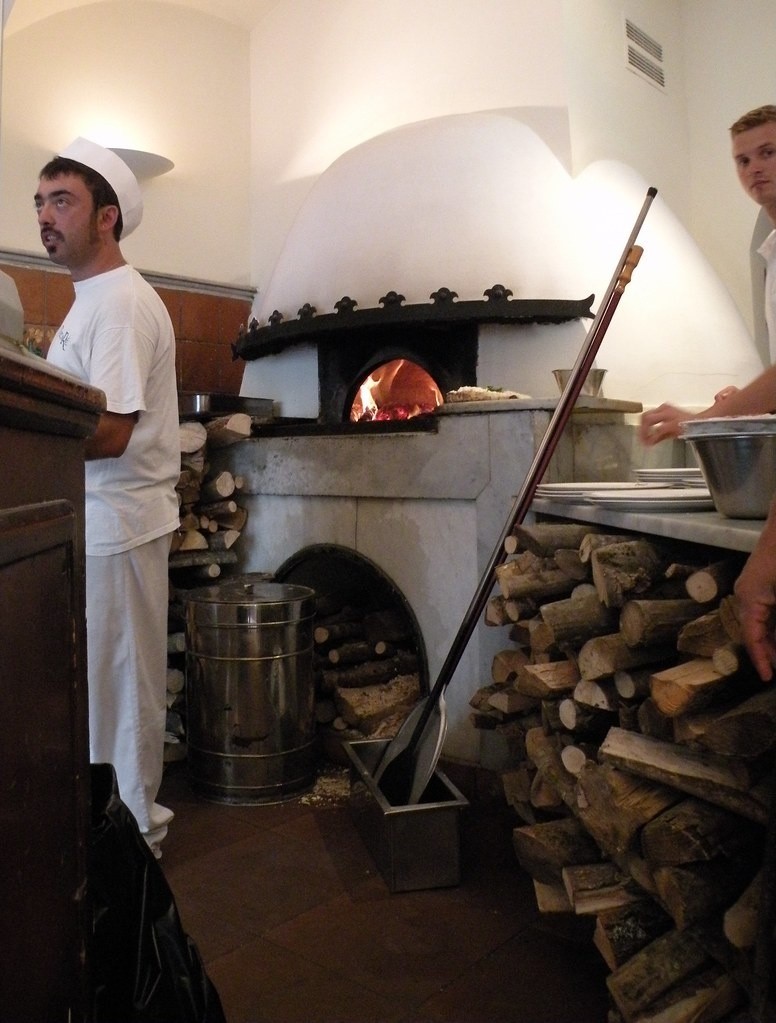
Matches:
[551,368,608,397]
[677,418,775,519]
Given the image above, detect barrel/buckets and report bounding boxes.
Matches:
[181,581,318,806]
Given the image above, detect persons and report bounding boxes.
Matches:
[733,493,776,682]
[34,135,182,859]
[639,104,776,450]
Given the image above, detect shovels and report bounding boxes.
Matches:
[372,184,663,813]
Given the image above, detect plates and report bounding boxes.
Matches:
[534,468,715,513]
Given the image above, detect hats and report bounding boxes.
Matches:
[55,138,144,241]
